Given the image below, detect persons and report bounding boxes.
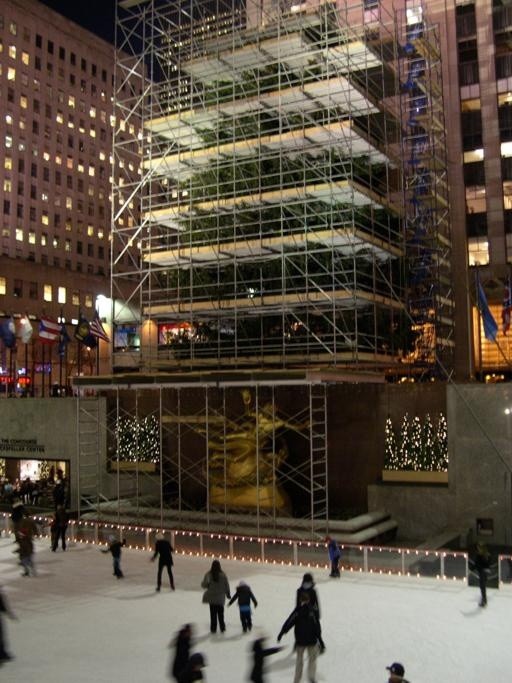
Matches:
[474,540,491,604]
[324,536,341,577]
[386,663,410,683]
[173,560,325,683]
[0,474,69,663]
[151,532,175,590]
[100,535,126,578]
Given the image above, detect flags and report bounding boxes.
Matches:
[501,275,511,338]
[74,318,96,347]
[18,315,33,344]
[38,315,62,344]
[58,322,71,355]
[89,312,110,343]
[2,317,15,347]
[477,275,498,342]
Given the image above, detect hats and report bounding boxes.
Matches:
[109,535,116,542]
[155,532,164,539]
[324,536,332,543]
[239,581,246,586]
[387,663,403,674]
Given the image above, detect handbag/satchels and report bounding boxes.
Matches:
[203,590,209,603]
